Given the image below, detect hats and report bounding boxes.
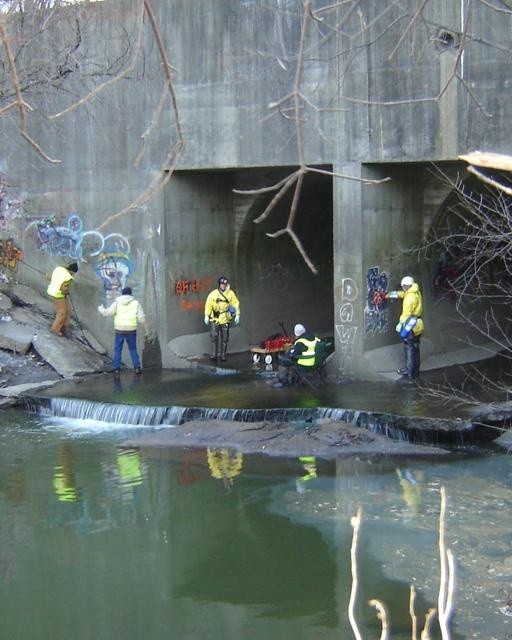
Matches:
[67,262,79,273]
[122,287,132,295]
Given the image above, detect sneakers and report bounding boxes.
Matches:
[135,368,142,374]
[114,369,121,374]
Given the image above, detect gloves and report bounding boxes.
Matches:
[204,316,210,326]
[395,322,402,333]
[234,314,241,326]
[97,303,106,314]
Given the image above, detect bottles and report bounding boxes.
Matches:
[230,307,236,314]
[399,318,417,338]
[404,466,417,486]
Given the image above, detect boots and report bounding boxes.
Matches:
[221,342,228,361]
[271,364,290,388]
[210,342,218,359]
[397,345,420,378]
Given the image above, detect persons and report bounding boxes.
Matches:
[97,286,149,374]
[391,466,426,524]
[202,276,241,361]
[373,275,424,377]
[51,444,83,535]
[105,446,150,505]
[272,324,320,389]
[205,446,244,497]
[287,454,321,497]
[45,263,78,338]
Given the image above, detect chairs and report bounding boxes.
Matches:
[291,339,334,392]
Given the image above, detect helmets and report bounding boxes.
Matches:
[400,276,414,286]
[293,323,307,337]
[218,276,229,284]
